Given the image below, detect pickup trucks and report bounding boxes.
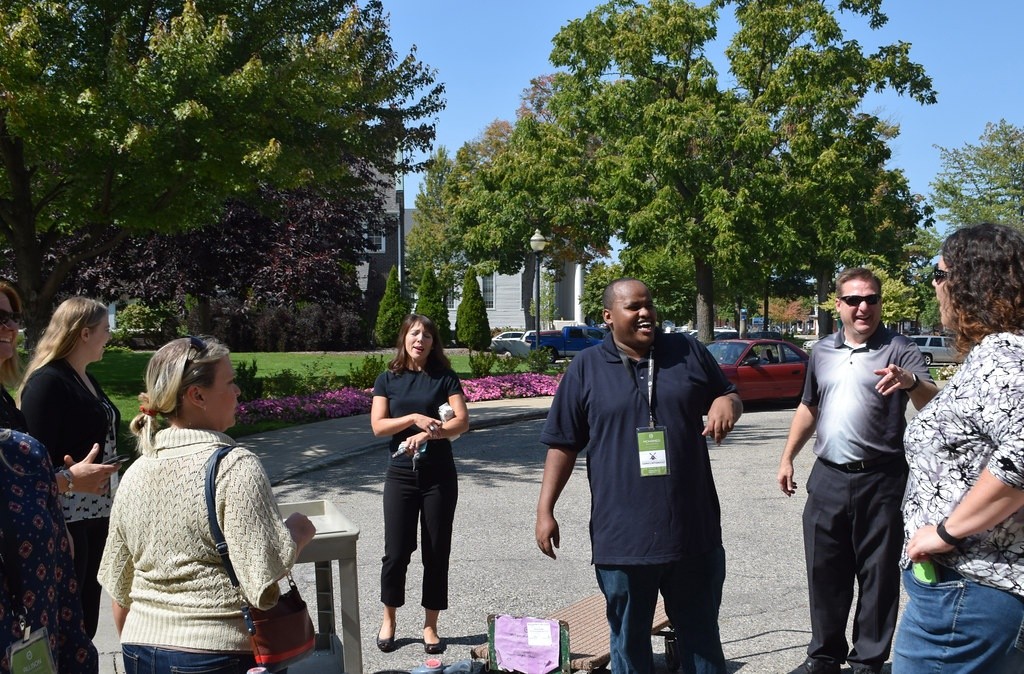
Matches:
[525,326,608,364]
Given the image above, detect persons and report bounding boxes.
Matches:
[371,314,470,653]
[536,278,743,674]
[777,268,942,674]
[0,284,122,674]
[892,223,1024,674]
[96,337,316,674]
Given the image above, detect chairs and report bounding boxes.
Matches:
[761,349,774,364]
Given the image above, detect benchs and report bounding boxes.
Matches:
[471,592,686,674]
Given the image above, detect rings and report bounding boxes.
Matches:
[99,481,104,489]
[409,444,415,448]
[430,426,434,431]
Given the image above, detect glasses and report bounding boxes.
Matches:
[183,336,208,373]
[0,309,23,326]
[837,294,882,307]
[934,263,951,283]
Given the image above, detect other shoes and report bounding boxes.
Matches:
[788,657,841,674]
[853,668,878,673]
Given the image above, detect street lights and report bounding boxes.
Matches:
[529,228,546,349]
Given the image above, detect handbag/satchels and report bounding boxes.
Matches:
[241,581,316,667]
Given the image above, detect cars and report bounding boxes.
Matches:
[489,330,562,359]
[704,339,809,401]
[661,325,790,339]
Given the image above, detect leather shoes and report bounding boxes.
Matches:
[423,633,442,653]
[376,621,396,651]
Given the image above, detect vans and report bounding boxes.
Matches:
[908,335,962,366]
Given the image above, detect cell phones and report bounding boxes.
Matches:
[912,559,938,583]
[102,454,130,465]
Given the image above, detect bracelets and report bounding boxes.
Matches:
[59,469,74,497]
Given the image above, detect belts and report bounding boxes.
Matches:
[819,457,894,471]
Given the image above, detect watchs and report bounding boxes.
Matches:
[936,517,967,546]
[905,374,920,392]
